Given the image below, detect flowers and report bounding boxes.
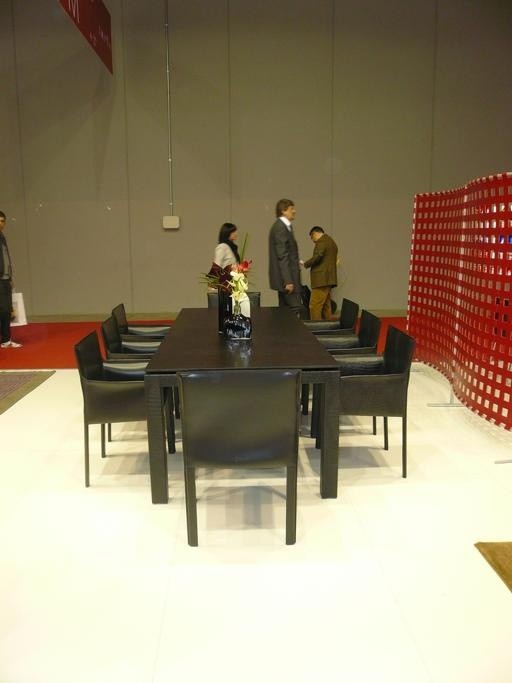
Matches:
[226,259,254,314]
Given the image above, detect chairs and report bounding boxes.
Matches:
[208,290,260,308]
[175,368,304,546]
[73,300,179,489]
[299,296,414,477]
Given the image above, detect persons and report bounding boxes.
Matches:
[300,226,338,321]
[213,223,250,304]
[0,211,23,348]
[268,199,304,306]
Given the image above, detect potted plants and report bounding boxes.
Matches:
[197,232,253,335]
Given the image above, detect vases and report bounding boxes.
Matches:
[223,312,253,340]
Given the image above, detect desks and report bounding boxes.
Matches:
[143,305,342,504]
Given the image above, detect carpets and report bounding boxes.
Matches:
[1,369,57,414]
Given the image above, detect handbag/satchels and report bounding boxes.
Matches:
[8,289,29,327]
